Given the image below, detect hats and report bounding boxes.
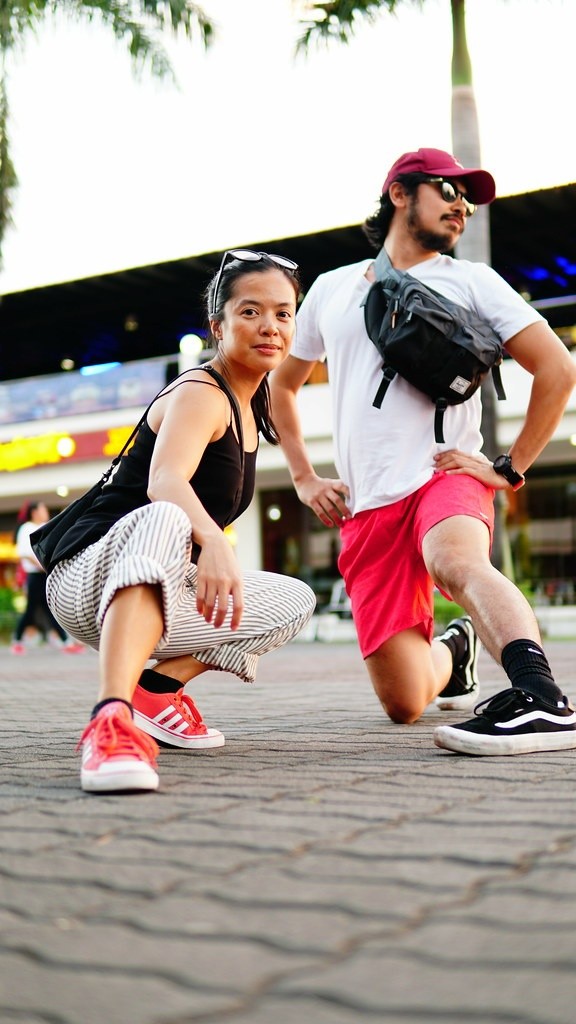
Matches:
[381,148,496,206]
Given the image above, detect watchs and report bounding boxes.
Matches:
[492,453,525,491]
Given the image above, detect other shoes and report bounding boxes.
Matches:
[62,643,84,655]
[12,641,27,656]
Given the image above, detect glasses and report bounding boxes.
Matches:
[408,177,479,217]
[213,249,300,314]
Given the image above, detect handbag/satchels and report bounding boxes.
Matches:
[359,246,507,444]
[29,471,110,576]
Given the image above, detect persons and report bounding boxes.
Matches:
[265,147,576,757]
[9,501,85,656]
[30,247,316,793]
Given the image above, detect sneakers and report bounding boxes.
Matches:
[433,687,576,755]
[434,614,481,711]
[75,683,226,791]
